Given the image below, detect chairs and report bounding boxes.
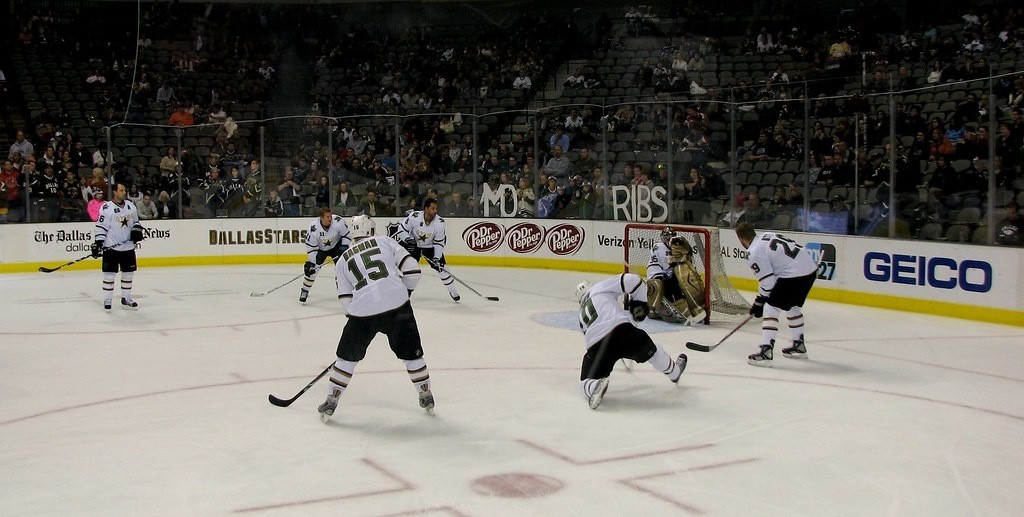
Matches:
[1,45,259,190]
[316,71,522,212]
[566,17,1023,246]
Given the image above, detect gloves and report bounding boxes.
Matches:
[431,257,444,272]
[130,225,143,244]
[91,243,103,259]
[406,237,418,255]
[304,262,316,277]
[750,295,769,318]
[630,299,649,321]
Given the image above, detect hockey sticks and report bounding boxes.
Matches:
[686,313,756,352]
[420,254,499,301]
[250,255,339,298]
[38,234,143,273]
[621,359,634,373]
[622,259,707,327]
[268,360,337,408]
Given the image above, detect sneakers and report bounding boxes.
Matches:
[122,297,138,312]
[300,290,308,304]
[782,334,807,359]
[104,299,112,311]
[589,378,609,409]
[418,383,435,416]
[748,339,775,367]
[450,292,460,303]
[318,388,343,422]
[672,354,687,383]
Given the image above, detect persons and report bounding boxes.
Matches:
[319,215,436,423]
[646,228,684,303]
[299,207,353,306]
[398,198,460,302]
[736,222,819,367]
[575,274,688,408]
[92,182,143,312]
[0,0,1024,248]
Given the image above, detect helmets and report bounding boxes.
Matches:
[660,227,676,242]
[575,280,593,302]
[350,214,376,238]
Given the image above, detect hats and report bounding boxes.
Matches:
[545,175,615,197]
